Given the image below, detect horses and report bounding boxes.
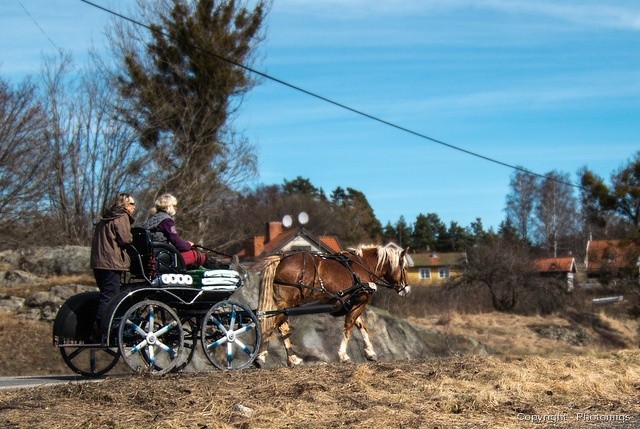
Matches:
[245,243,413,365]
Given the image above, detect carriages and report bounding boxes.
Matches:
[53,226,337,380]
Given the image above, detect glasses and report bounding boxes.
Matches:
[130,203,136,206]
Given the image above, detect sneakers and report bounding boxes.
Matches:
[228,254,239,270]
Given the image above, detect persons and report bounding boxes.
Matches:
[89,192,136,344]
[142,193,239,270]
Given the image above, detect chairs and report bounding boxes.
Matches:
[128,226,186,285]
